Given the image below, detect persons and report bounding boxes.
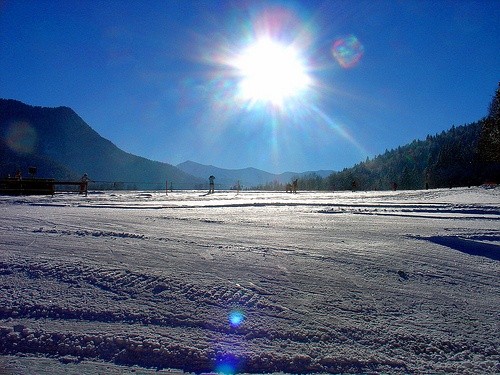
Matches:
[208,175,215,193]
[351,179,356,191]
[285,178,297,193]
[79,173,96,194]
[15,168,24,184]
